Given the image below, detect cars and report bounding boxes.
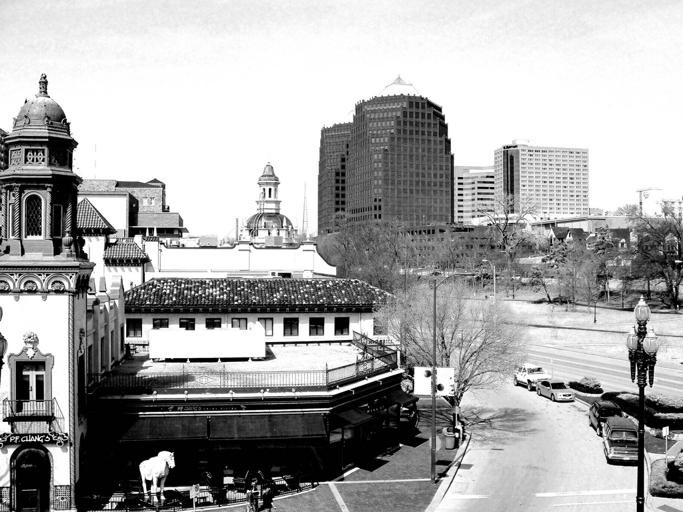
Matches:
[513,363,576,401]
[590,400,639,463]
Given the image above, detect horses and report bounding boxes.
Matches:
[138,450,177,494]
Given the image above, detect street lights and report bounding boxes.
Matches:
[626,294,657,512]
[423,273,475,484]
[482,259,496,301]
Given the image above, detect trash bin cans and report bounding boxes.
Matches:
[0,487,13,512]
[55,485,72,511]
[444,432,456,451]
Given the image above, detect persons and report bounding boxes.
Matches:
[246,488,273,512]
